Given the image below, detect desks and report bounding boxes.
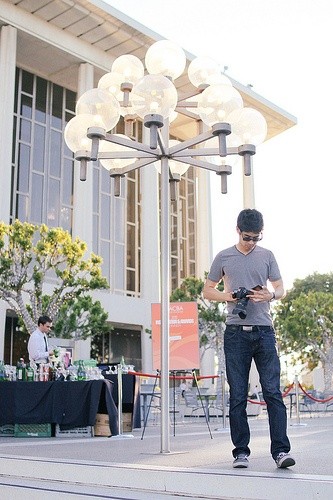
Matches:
[139,392,160,427]
[0,379,118,438]
[103,374,141,429]
[287,393,296,418]
[195,394,217,423]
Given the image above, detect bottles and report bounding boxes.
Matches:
[0,348,86,382]
[120,356,124,368]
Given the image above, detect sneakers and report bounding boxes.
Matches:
[232,452,250,468]
[275,452,296,468]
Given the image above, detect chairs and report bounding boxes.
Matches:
[296,392,333,418]
[148,387,207,427]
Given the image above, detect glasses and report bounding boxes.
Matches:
[240,230,263,241]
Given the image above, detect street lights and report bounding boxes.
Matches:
[63,41,268,454]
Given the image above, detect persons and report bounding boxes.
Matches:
[203,209,294,468]
[28,315,53,370]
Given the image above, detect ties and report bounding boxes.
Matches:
[44,336,48,363]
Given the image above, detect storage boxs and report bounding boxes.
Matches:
[0,413,132,438]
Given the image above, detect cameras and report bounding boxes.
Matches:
[231,285,263,319]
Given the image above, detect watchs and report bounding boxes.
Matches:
[268,292,275,302]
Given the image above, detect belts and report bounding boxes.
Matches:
[227,324,274,333]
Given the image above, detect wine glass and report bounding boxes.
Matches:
[63,366,105,382]
[107,365,135,374]
